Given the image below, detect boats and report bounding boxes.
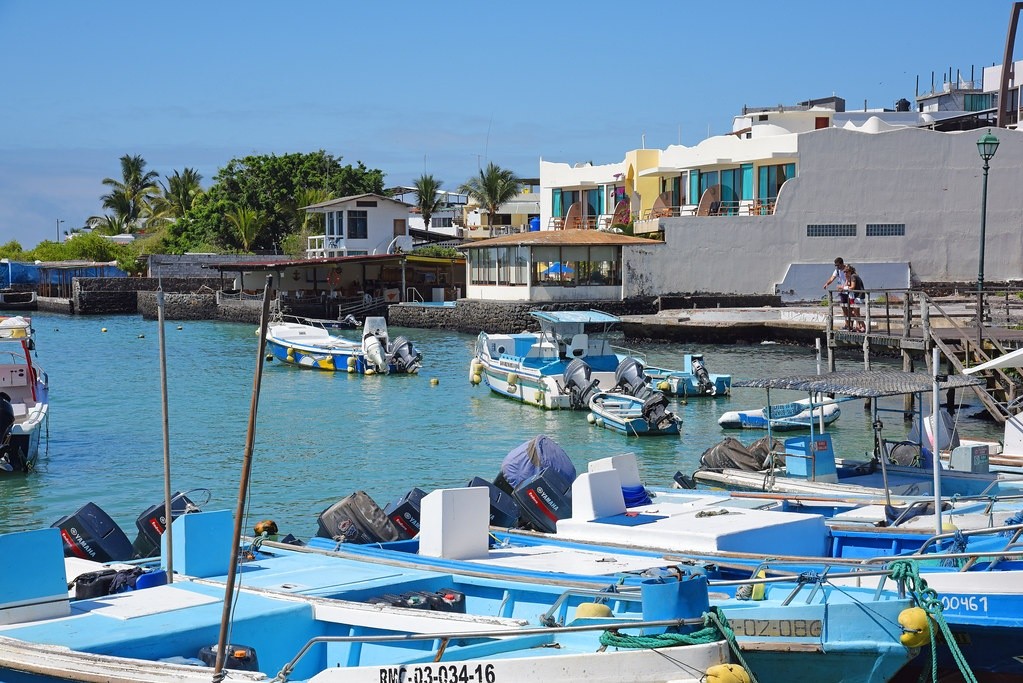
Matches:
[474,308,648,409]
[0,316,50,475]
[257,322,425,374]
[0,376,1023,683]
[643,367,731,397]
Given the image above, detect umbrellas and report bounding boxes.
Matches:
[540,263,574,280]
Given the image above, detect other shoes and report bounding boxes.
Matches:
[850,327,859,332]
[842,325,848,329]
[859,327,865,333]
[847,326,853,330]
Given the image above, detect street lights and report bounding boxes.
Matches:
[56,221,64,241]
[975,129,1000,291]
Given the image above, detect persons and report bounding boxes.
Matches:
[823,257,854,332]
[352,280,361,291]
[839,264,867,332]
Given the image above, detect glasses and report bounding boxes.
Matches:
[843,270,849,273]
[835,264,841,267]
